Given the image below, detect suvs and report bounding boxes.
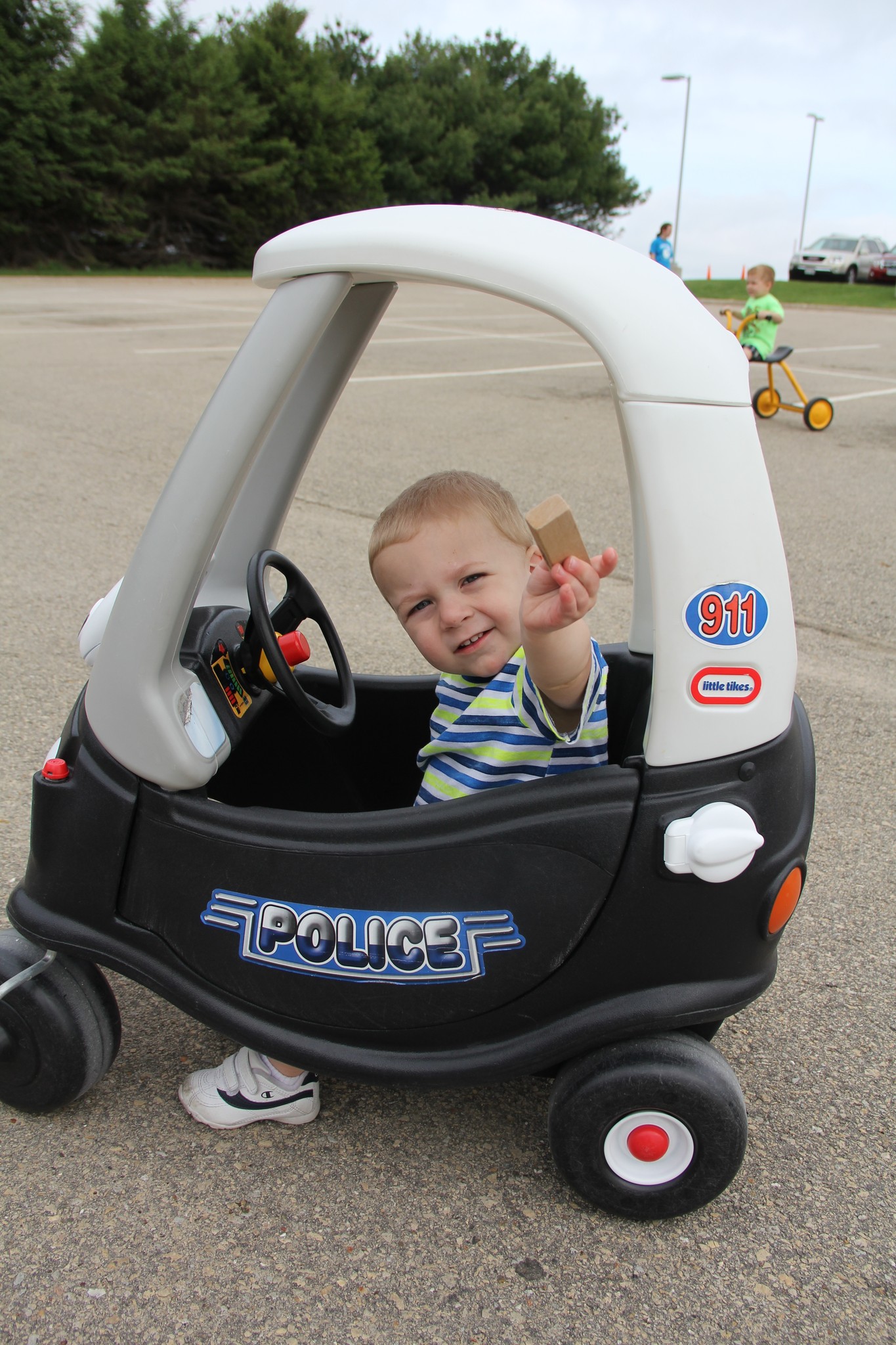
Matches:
[788,233,896,285]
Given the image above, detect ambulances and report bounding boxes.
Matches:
[0,206,817,1221]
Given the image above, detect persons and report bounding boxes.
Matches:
[649,222,675,271]
[722,264,785,360]
[178,470,617,1129]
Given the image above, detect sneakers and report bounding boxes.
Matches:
[178,1047,321,1130]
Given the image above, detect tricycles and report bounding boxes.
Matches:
[718,309,835,431]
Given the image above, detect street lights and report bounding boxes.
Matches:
[661,75,691,278]
[797,111,824,249]
